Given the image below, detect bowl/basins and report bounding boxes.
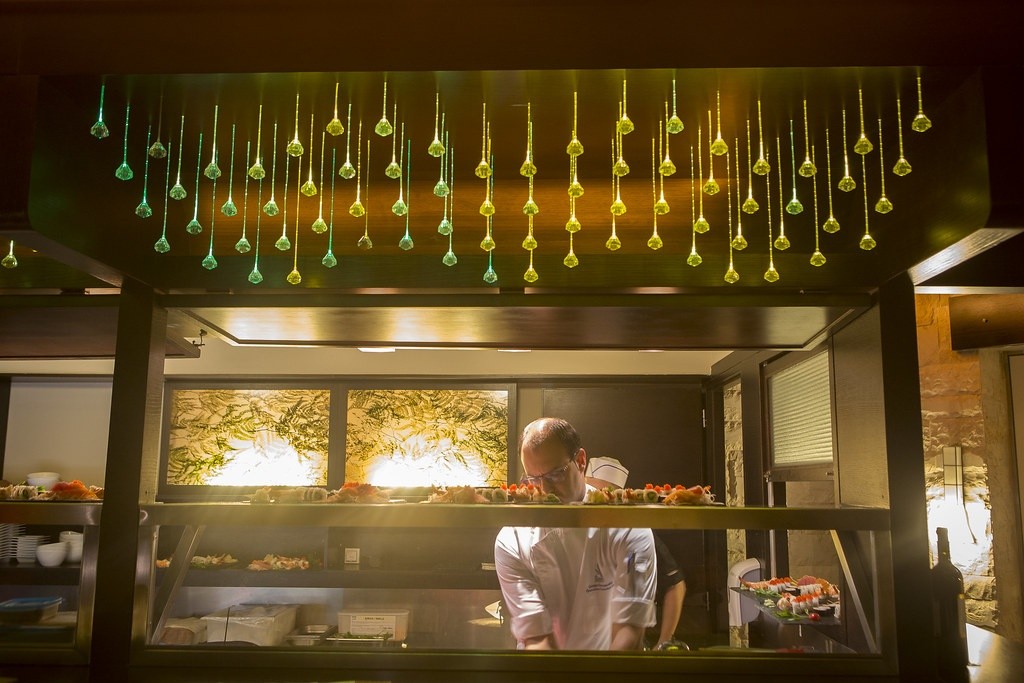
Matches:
[35,530,84,567]
[26,471,62,488]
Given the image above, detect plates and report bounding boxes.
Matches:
[0,522,51,563]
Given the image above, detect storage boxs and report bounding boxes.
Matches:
[205,602,300,647]
[337,607,410,643]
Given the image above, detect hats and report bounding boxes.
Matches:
[585,457,628,488]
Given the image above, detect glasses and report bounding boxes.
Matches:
[518,455,575,484]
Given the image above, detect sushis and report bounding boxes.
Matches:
[250,481,715,505]
[766,574,840,615]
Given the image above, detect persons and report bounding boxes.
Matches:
[494,417,658,653]
[584,457,686,651]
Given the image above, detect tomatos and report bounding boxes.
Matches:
[809,613,820,621]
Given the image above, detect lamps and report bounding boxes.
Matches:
[942,447,964,507]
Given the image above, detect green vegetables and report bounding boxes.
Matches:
[755,587,810,620]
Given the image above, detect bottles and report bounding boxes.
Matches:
[931,526,966,667]
